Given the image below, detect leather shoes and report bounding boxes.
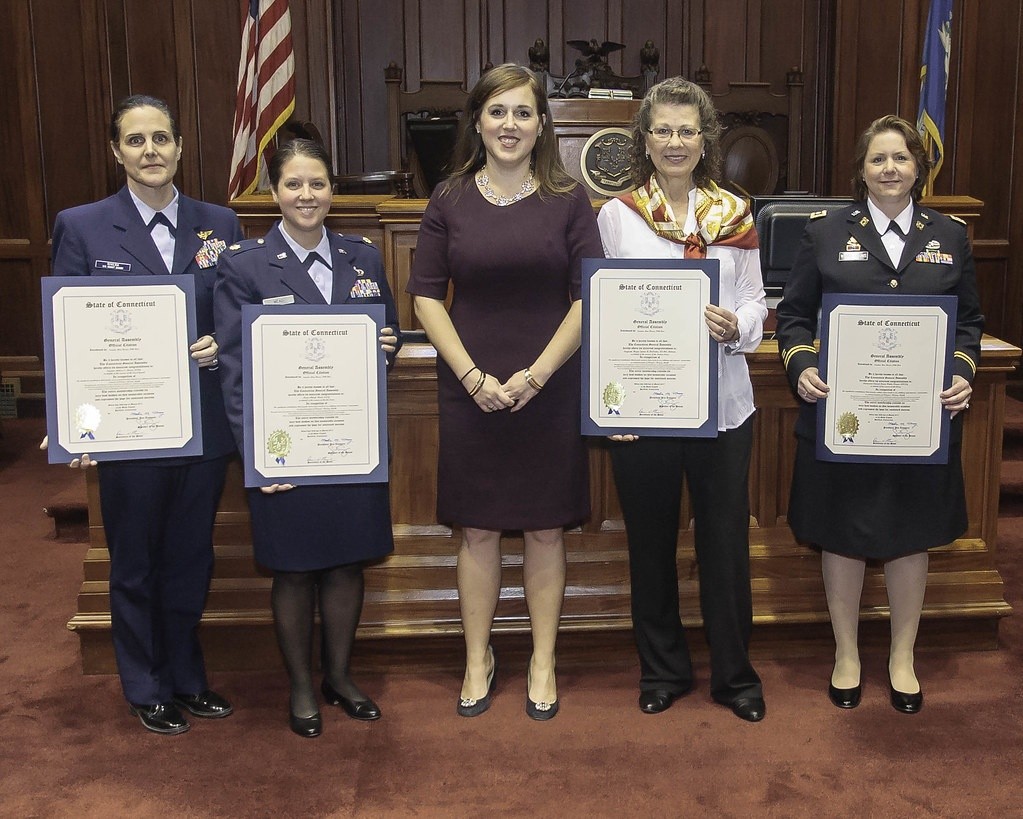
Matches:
[289,695,321,737]
[322,677,381,719]
[829,662,862,708]
[887,654,923,713]
[164,689,233,718]
[129,703,190,734]
[639,690,672,713]
[731,697,766,722]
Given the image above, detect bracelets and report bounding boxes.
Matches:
[467,373,490,397]
[460,366,479,381]
[525,370,544,390]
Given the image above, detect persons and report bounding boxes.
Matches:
[775,110,984,714]
[214,138,403,738]
[52,95,241,735]
[408,64,616,720]
[596,76,769,722]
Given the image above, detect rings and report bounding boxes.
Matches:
[211,358,218,366]
[721,327,726,337]
[803,390,808,398]
[964,402,970,410]
[490,405,494,409]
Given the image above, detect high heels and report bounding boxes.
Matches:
[526,660,558,721]
[458,644,496,716]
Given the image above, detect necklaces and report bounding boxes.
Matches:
[478,165,534,206]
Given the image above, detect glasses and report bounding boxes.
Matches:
[645,127,704,140]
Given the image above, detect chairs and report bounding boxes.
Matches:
[696,62,805,195]
[382,59,496,197]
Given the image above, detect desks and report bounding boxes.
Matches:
[66,169,1023,675]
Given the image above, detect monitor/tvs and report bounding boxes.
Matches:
[750,191,856,287]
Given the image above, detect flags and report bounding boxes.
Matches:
[917,0,952,198]
[227,0,295,201]
